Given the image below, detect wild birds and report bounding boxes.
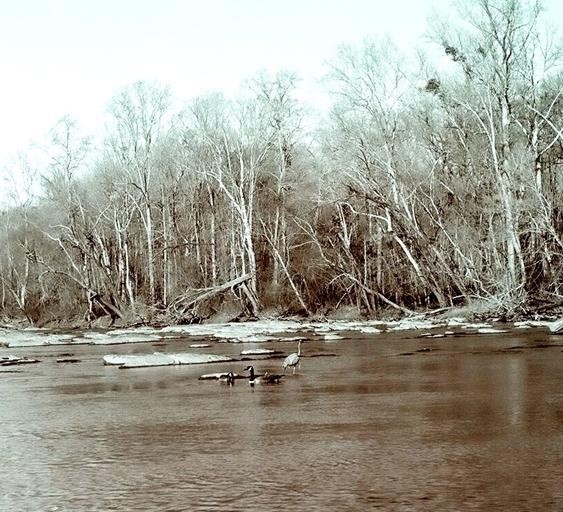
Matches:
[281,339,312,374]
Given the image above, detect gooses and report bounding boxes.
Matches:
[227,372,235,387]
[243,366,288,386]
